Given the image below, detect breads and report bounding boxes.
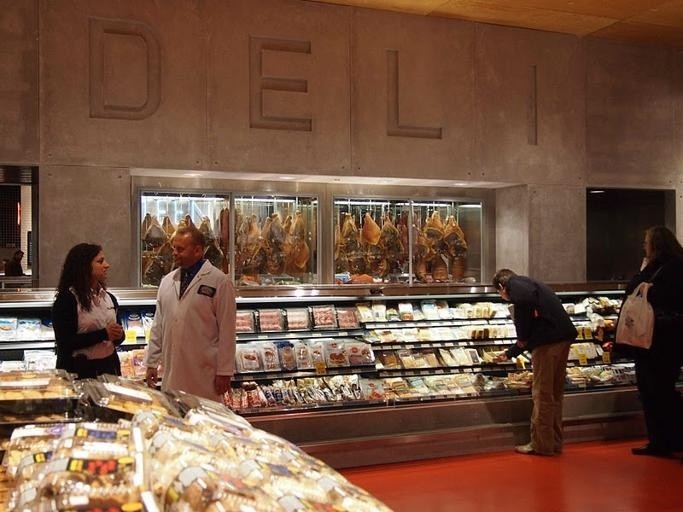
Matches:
[0,370,394,511]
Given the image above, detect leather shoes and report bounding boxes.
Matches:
[631,443,670,455]
[514,442,539,454]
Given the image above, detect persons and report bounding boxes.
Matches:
[607,225,682,461]
[49,241,124,379]
[490,266,581,457]
[3,248,26,277]
[143,224,238,405]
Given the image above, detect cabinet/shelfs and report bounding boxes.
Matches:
[0,293,683,470]
